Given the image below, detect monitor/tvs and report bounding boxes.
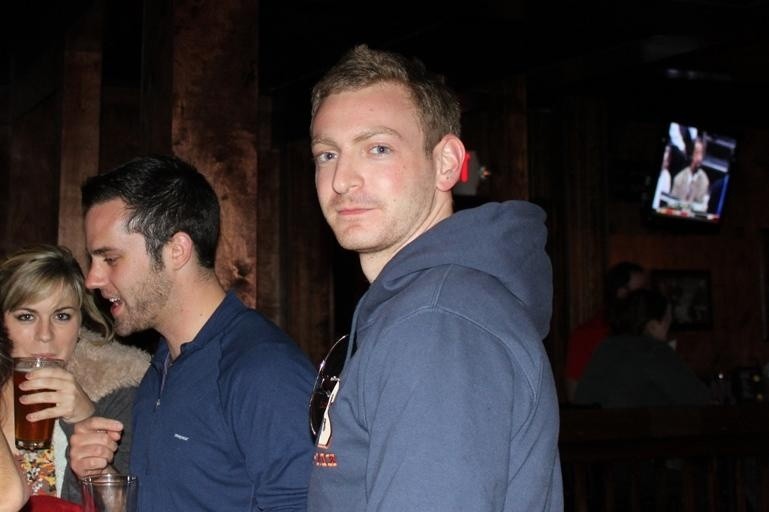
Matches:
[637,113,742,236]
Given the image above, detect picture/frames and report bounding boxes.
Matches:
[649,266,715,333]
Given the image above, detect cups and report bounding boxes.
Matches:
[82,475,136,509]
[12,355,64,448]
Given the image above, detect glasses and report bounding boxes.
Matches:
[308,335,349,443]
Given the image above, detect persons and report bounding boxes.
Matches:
[652,147,672,210]
[0,242,151,511]
[80,152,322,511]
[583,285,709,510]
[307,43,566,512]
[673,139,712,214]
[563,263,648,408]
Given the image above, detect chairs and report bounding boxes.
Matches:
[566,406,708,512]
